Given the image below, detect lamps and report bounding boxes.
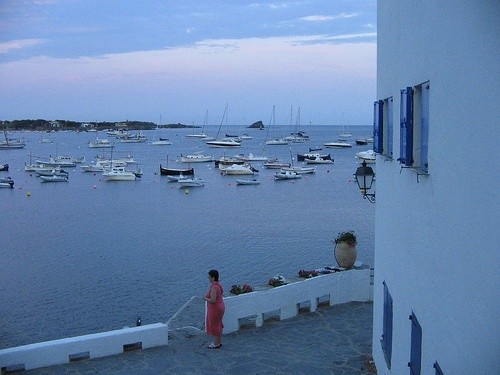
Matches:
[353,159,375,204]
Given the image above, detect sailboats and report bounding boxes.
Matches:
[338,117,351,140]
[152,114,172,145]
[283,105,310,143]
[265,104,288,145]
[205,102,242,147]
[38,123,54,143]
[185,110,208,138]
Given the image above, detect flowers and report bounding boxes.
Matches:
[230,284,253,295]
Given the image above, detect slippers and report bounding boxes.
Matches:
[208,343,223,348]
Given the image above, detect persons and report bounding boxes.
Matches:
[204,270,225,348]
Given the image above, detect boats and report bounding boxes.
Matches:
[202,136,216,141]
[295,152,334,164]
[364,138,373,143]
[213,152,316,179]
[355,138,368,145]
[159,164,194,177]
[164,174,205,189]
[234,179,261,185]
[179,152,217,163]
[222,129,253,143]
[24,141,86,182]
[354,148,376,164]
[88,128,148,148]
[0,164,9,171]
[0,127,26,150]
[322,141,351,149]
[0,176,15,189]
[78,143,143,181]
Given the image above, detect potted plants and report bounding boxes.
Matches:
[331,230,357,266]
